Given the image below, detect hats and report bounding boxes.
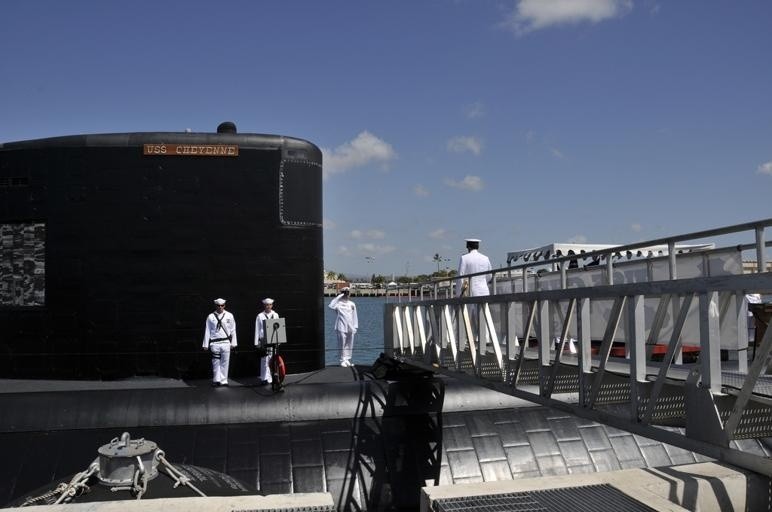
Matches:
[214,298,226,304]
[464,239,481,248]
[262,298,274,304]
[340,287,350,291]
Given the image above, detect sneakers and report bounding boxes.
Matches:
[212,382,229,387]
[261,380,267,384]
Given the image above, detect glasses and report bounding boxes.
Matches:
[217,305,224,307]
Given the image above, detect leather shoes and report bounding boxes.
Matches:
[340,364,354,367]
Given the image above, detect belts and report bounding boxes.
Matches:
[209,338,228,342]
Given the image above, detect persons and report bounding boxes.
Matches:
[202,298,238,386]
[328,287,358,368]
[454,238,492,296]
[254,298,279,385]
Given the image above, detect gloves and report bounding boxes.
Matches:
[337,294,344,299]
